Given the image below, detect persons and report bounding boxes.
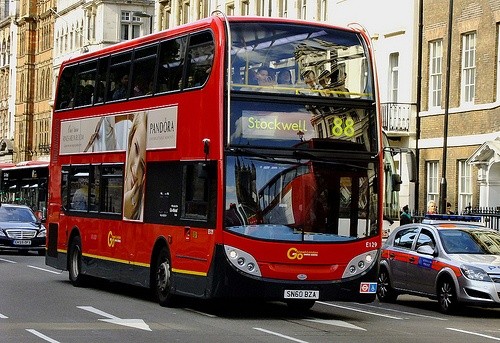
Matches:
[446,201,456,215]
[427,199,439,215]
[123,110,150,220]
[399,205,414,227]
[63,62,349,106]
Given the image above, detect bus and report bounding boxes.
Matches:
[381,128,418,246]
[45,9,402,311]
[0,160,49,226]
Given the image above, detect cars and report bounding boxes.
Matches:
[377,224,500,316]
[0,203,46,255]
